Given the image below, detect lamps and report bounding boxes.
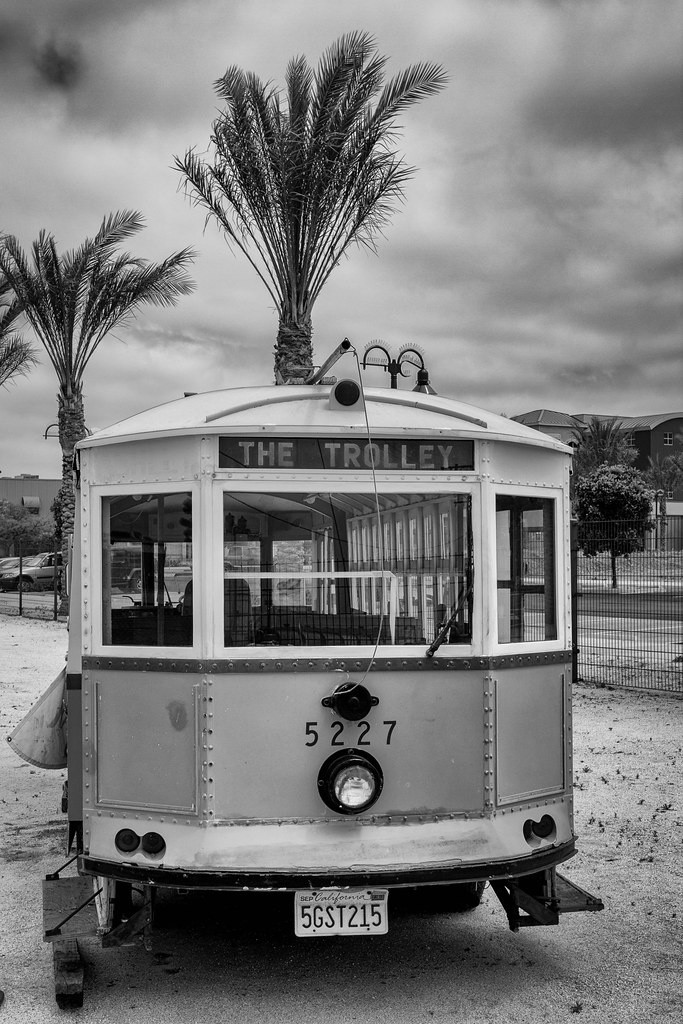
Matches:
[303,493,316,504]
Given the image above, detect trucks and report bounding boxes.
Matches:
[225,545,279,571]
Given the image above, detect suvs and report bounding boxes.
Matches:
[57,545,158,595]
[0,552,62,592]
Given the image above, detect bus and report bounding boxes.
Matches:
[5,340,603,953]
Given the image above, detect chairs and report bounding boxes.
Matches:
[182,578,253,648]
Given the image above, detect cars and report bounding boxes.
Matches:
[1,558,55,571]
[0,557,22,568]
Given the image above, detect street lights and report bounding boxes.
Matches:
[650,490,665,552]
[357,341,438,396]
